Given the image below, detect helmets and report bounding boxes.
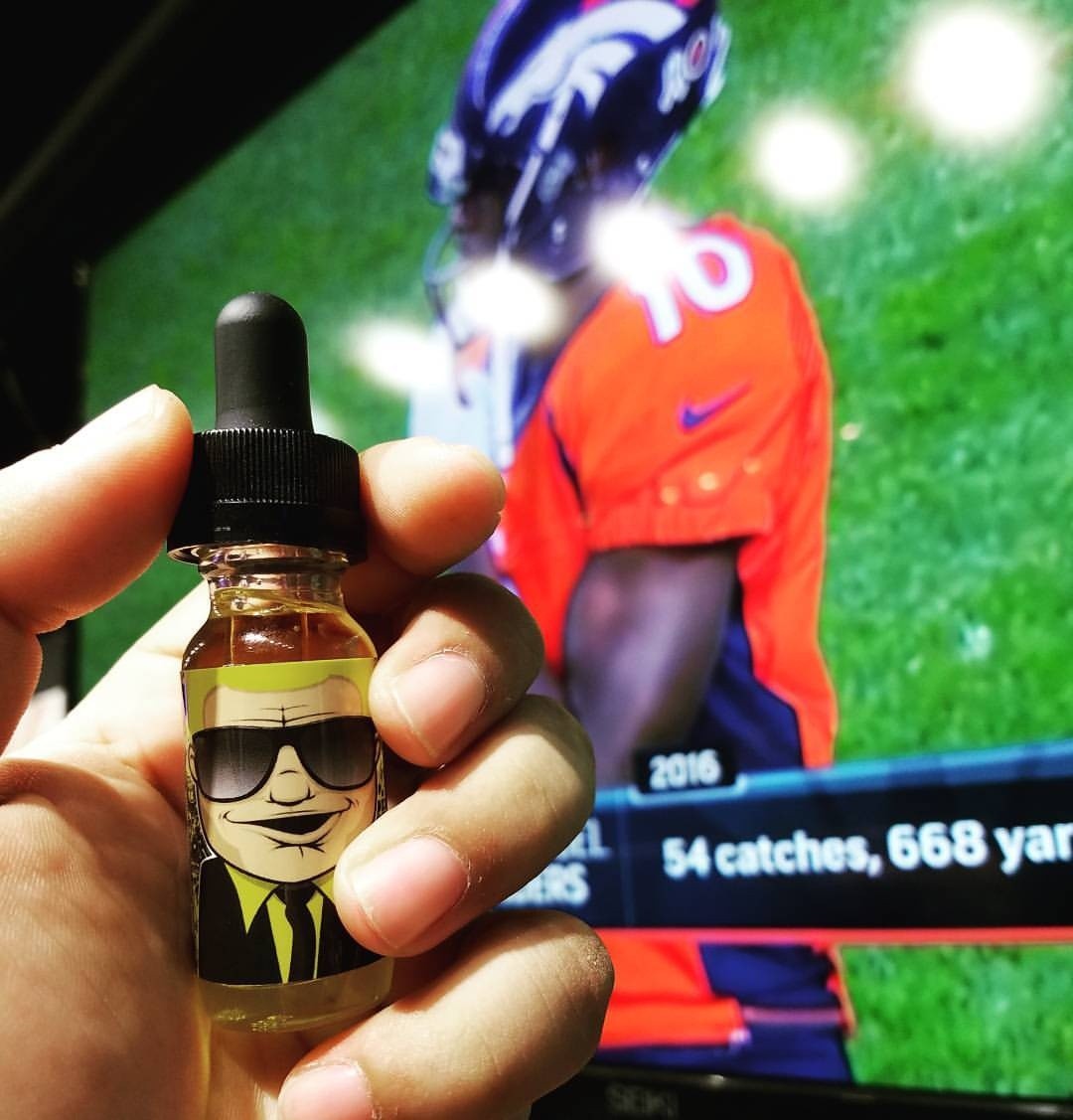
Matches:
[428,0,723,271]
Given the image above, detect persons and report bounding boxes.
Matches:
[0,386,614,1120]
[408,2,861,1087]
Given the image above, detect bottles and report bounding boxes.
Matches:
[163,293,395,1036]
